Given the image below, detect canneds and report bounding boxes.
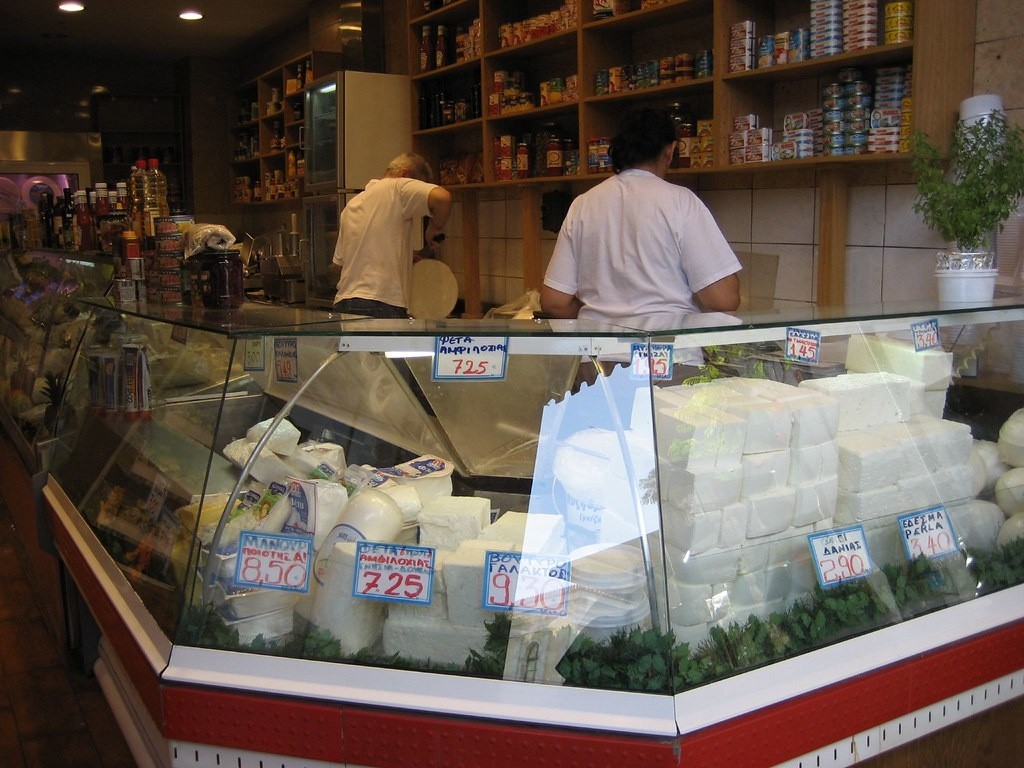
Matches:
[487,0,913,181]
[454,101,466,123]
[198,248,244,309]
[112,214,196,305]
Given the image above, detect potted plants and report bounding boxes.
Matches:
[908,109,1023,268]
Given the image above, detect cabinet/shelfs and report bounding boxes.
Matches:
[406,0,977,190]
[226,45,342,205]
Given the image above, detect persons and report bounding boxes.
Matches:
[328,153,452,467]
[539,108,742,394]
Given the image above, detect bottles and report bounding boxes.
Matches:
[294,103,302,120]
[13,181,128,252]
[418,24,481,128]
[515,121,577,178]
[270,120,282,153]
[129,158,170,237]
[289,150,305,175]
[253,169,285,200]
[664,99,695,168]
[297,60,311,90]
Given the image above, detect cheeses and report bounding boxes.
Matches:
[380,335,1024,675]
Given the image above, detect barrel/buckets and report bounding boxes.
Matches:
[553,432,654,648]
